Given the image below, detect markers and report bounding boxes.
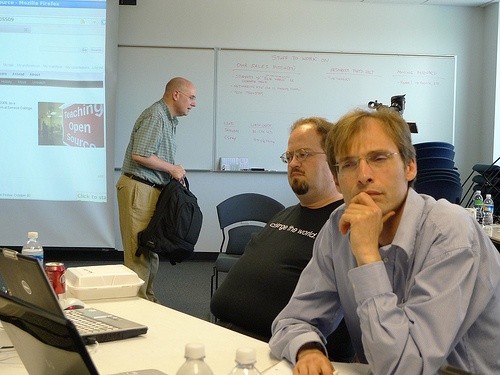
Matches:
[250,168,264,171]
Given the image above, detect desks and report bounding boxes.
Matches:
[81,296,280,375]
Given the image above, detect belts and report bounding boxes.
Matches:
[123,172,163,189]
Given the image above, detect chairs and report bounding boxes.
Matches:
[413,139,500,226]
[210,192,286,300]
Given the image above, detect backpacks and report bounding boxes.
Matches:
[135,177,203,265]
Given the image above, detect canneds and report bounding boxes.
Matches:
[45,262,66,295]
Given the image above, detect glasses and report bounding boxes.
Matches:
[178,91,196,100]
[338,151,402,174]
[280,147,327,164]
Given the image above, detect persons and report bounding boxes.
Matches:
[207,117,357,364]
[117,77,197,304]
[268,109,500,375]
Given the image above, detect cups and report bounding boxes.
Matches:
[464,207,476,220]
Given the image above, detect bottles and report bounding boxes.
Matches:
[21,231,43,267]
[473,190,484,225]
[174,344,214,375]
[482,194,494,226]
[229,346,261,375]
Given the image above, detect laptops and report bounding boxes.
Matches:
[0,247,148,344]
[0,298,166,375]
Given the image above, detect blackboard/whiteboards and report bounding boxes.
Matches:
[114,44,457,175]
